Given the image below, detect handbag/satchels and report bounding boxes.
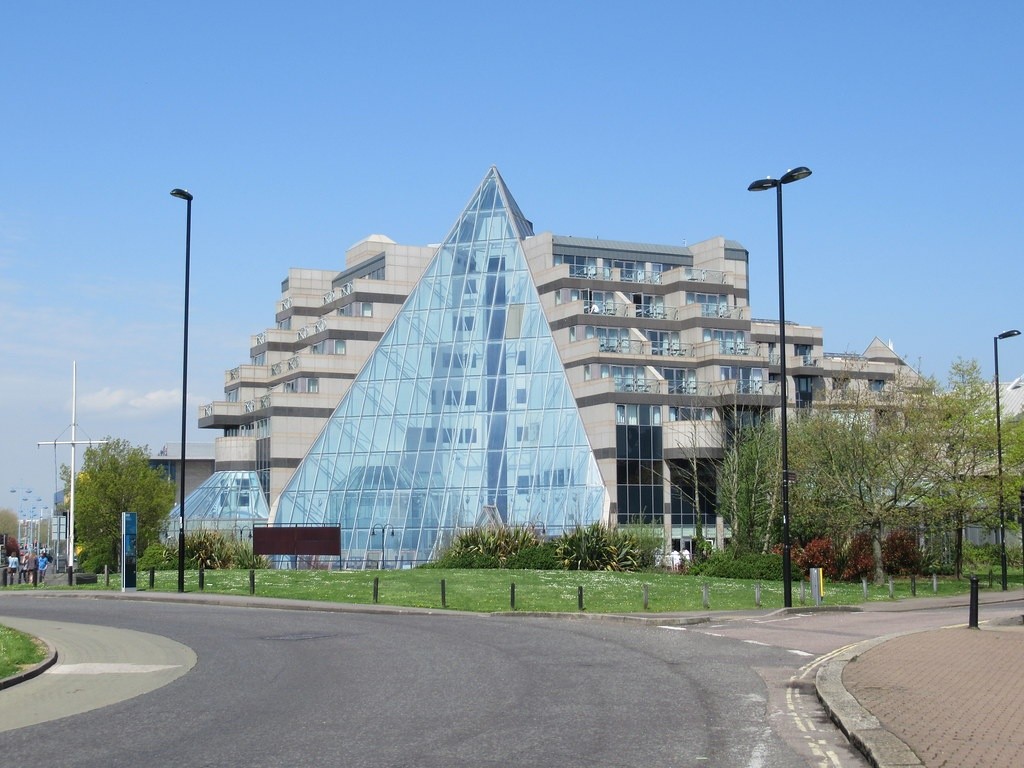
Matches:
[8,567,12,573]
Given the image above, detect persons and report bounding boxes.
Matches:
[653,547,691,571]
[7,552,49,585]
